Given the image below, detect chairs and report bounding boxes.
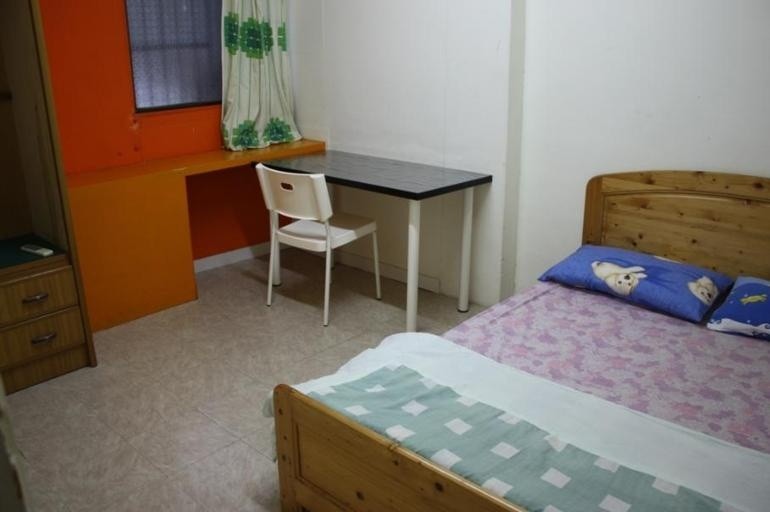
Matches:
[255,163,382,327]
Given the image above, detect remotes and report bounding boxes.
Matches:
[21,244,53,258]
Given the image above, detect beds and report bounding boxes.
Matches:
[274,170,769,512]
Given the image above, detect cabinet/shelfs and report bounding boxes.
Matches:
[0,231,98,396]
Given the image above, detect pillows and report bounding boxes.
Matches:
[538,242,770,340]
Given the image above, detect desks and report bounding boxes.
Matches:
[251,150,492,333]
[63,139,325,333]
